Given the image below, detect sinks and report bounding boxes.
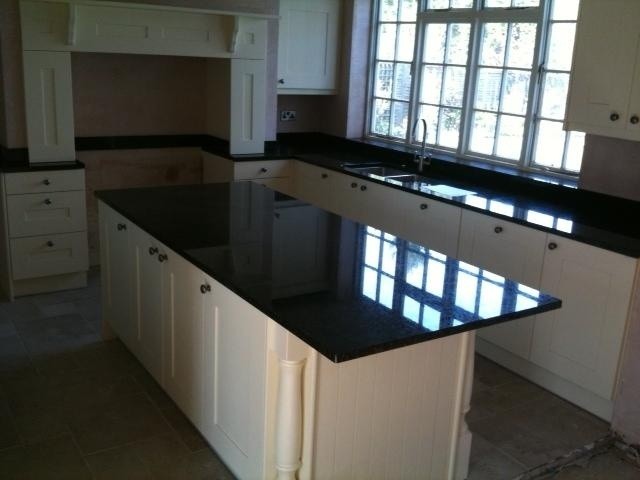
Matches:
[337,160,416,179]
[383,175,441,191]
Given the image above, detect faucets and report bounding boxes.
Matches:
[408,117,427,173]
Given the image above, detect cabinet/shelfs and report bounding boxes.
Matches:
[562,0,639,148]
[3,167,89,304]
[299,159,637,426]
[201,150,296,199]
[98,197,476,480]
